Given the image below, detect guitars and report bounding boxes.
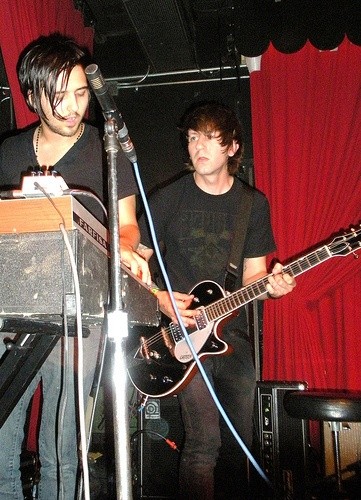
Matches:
[121,222,361,397]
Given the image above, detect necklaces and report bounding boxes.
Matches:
[36,123,83,167]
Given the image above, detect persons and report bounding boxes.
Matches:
[132,102,298,500]
[0,31,181,500]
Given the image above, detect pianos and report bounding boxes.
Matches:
[1,196,163,500]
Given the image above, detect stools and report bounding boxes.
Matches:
[283,389,361,500]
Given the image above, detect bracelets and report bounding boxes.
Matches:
[152,289,158,295]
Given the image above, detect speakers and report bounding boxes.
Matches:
[245,379,311,500]
[138,393,187,500]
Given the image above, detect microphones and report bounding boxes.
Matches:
[86,63,136,163]
